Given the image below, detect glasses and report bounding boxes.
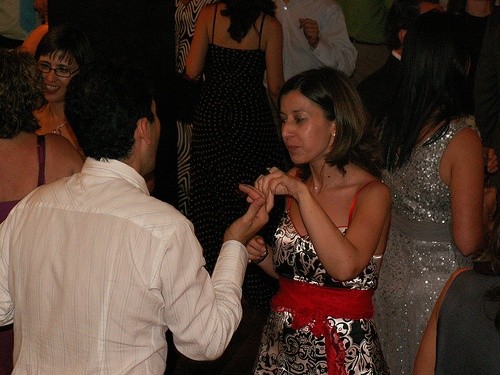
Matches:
[37,63,81,78]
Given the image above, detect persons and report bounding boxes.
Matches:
[350,0,500,189]
[0,58,274,375]
[367,9,500,375]
[413,183,500,375]
[184,0,293,316]
[262,0,359,90]
[246,66,392,375]
[0,0,157,374]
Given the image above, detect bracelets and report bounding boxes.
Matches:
[255,243,268,266]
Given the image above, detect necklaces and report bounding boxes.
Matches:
[47,109,69,138]
